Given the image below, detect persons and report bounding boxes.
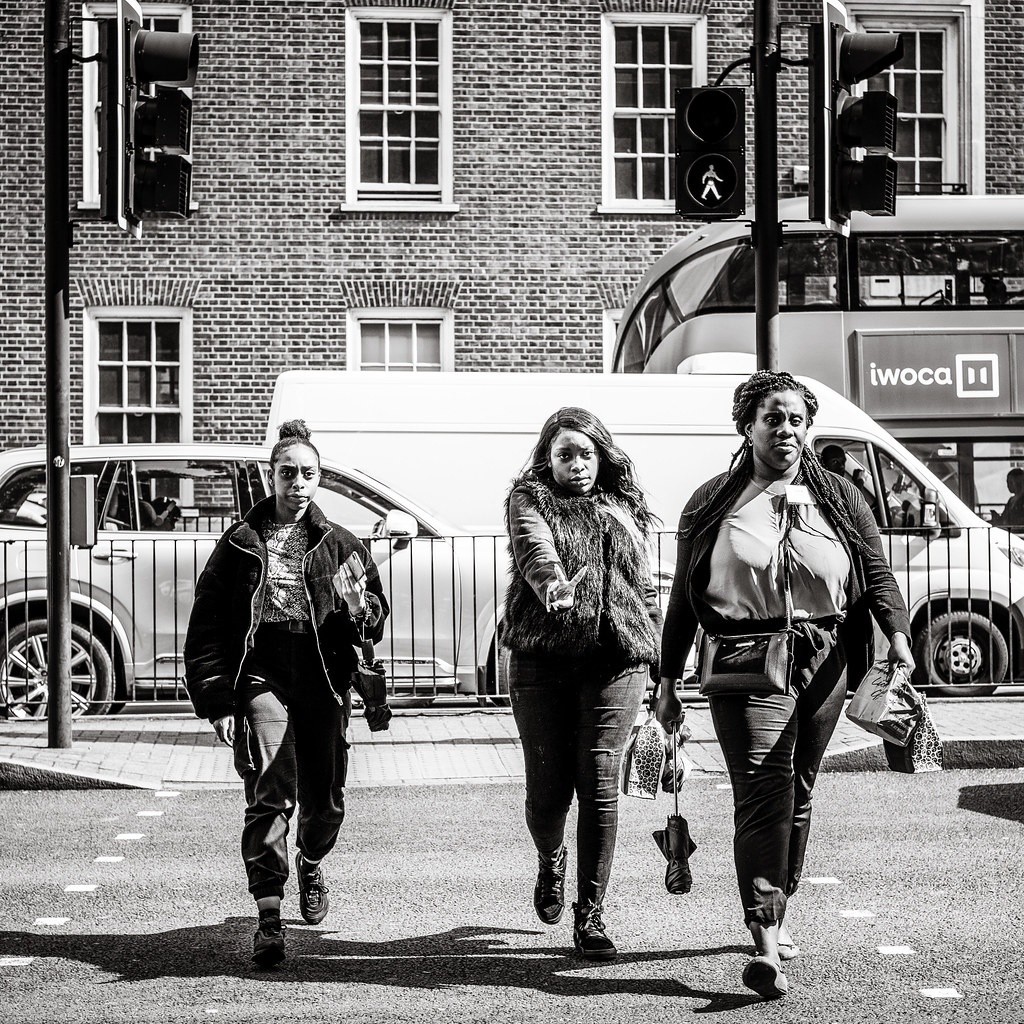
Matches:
[148,496,182,531]
[184,420,389,963]
[982,278,1007,305]
[654,368,916,997]
[495,408,665,959]
[990,465,1023,534]
[821,444,867,491]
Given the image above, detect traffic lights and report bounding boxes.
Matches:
[673,87,745,222]
[117,0,201,241]
[828,21,904,227]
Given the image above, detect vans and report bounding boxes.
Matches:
[264,371,1024,698]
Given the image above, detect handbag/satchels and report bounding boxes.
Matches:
[622,711,664,799]
[883,691,945,774]
[844,660,921,747]
[698,629,796,697]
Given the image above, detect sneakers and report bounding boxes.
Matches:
[570,898,616,960]
[252,915,287,964]
[533,847,567,924]
[295,851,329,924]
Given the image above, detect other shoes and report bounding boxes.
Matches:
[778,938,797,961]
[741,955,789,998]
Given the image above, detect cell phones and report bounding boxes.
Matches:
[332,551,365,599]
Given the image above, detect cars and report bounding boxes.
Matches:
[0,442,698,720]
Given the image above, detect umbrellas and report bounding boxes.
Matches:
[651,711,698,894]
[350,614,393,732]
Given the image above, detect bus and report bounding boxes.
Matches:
[611,182,1024,547]
